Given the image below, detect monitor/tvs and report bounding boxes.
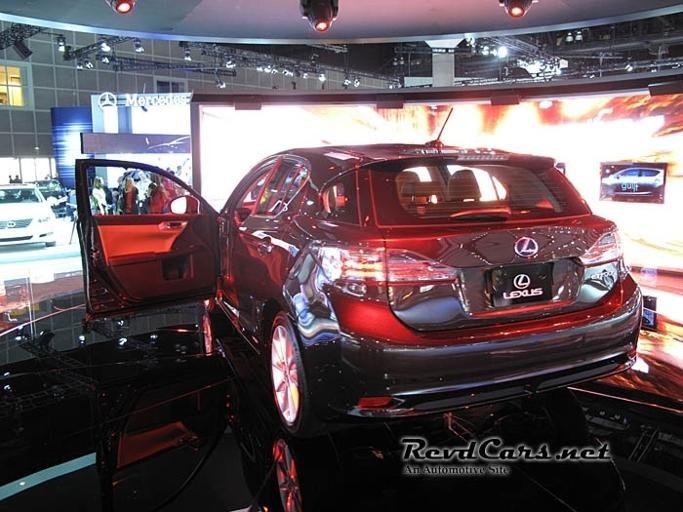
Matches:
[600,161,667,204]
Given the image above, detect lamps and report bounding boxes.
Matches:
[1,21,402,89]
[498,0,533,18]
[300,1,339,32]
[466,36,570,81]
[106,1,136,14]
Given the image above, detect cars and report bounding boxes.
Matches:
[1,180,72,247]
[601,166,665,195]
[75,139,644,440]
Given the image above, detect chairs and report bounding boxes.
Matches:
[396,170,504,214]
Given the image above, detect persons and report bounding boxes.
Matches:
[89,165,191,215]
[9,174,22,183]
[66,190,76,221]
